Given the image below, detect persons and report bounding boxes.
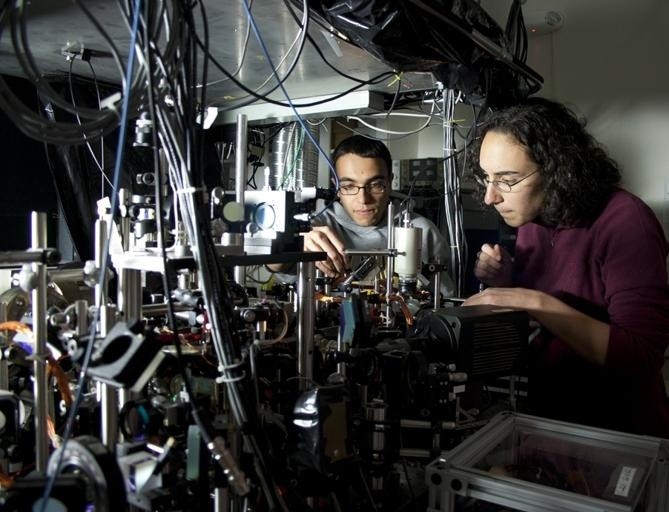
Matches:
[447,96,669,510]
[261,131,455,312]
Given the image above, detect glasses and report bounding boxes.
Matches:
[474,166,546,193]
[338,179,390,195]
